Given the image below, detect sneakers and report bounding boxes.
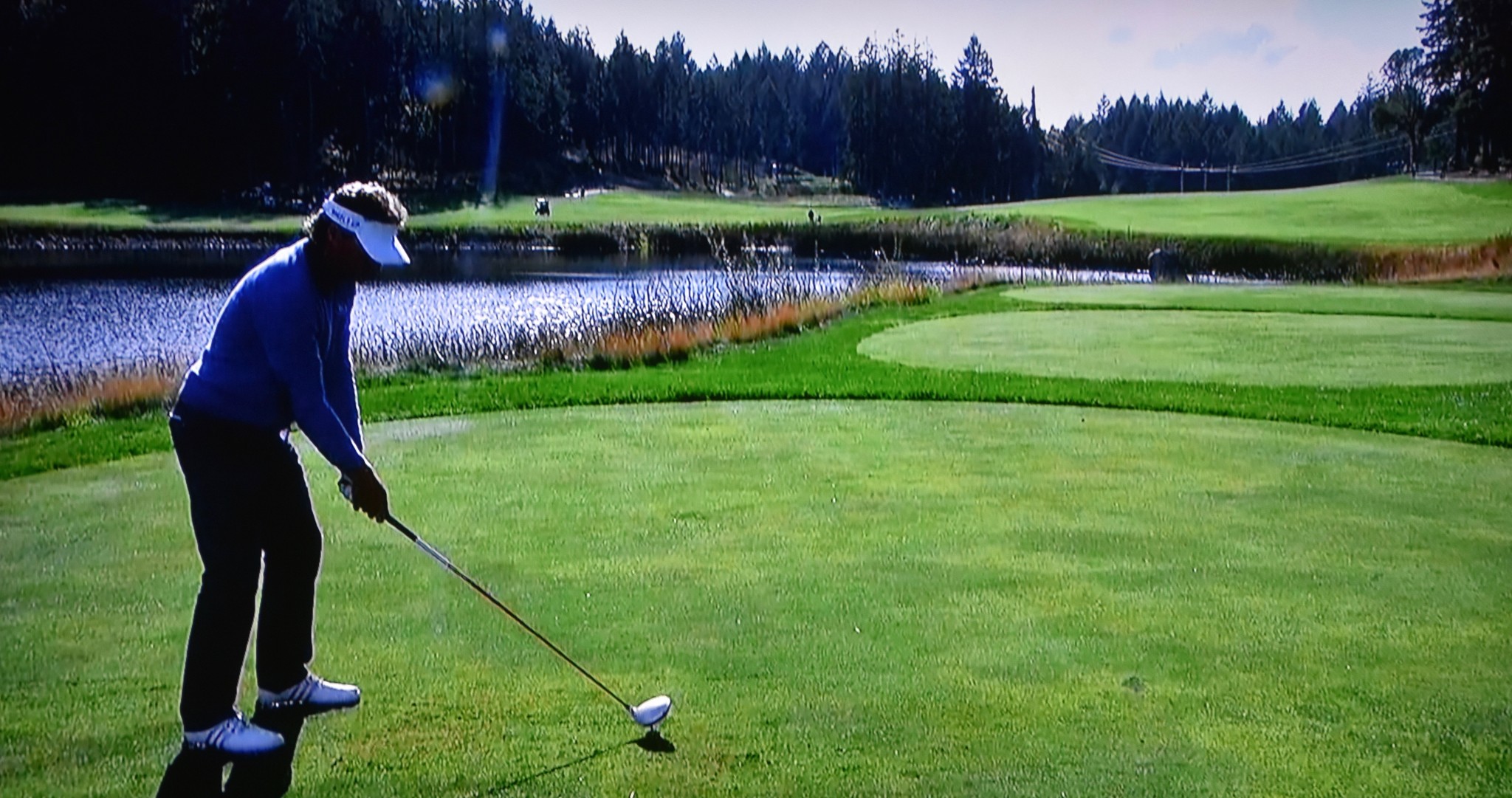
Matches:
[254,667,360,712]
[182,714,285,754]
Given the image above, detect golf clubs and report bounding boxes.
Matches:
[337,477,673,727]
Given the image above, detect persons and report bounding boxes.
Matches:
[165,181,412,755]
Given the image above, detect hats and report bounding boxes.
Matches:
[324,192,409,268]
